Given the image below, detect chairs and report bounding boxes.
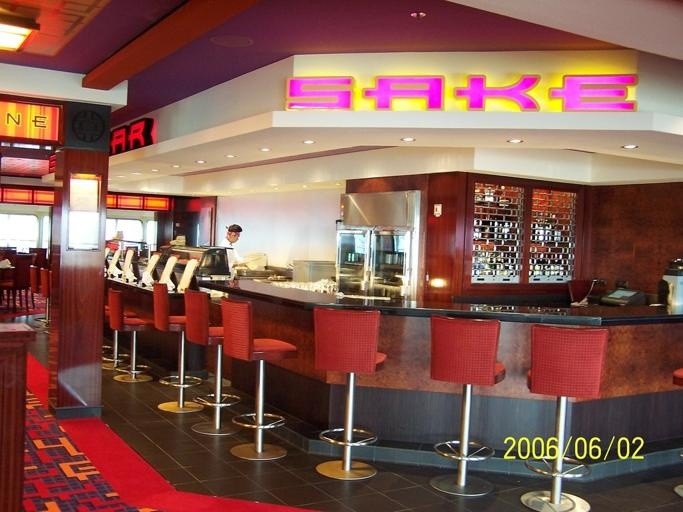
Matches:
[520,324,609,512]
[184,288,246,437]
[0,247,154,384]
[313,307,387,481]
[431,315,501,497]
[152,284,205,414]
[672,368,683,497]
[221,299,297,461]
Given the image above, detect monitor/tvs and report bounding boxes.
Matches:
[601,287,642,305]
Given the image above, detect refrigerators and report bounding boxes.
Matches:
[336,188,422,301]
[338,298,423,311]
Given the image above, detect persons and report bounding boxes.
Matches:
[220,224,242,272]
[112,230,124,250]
